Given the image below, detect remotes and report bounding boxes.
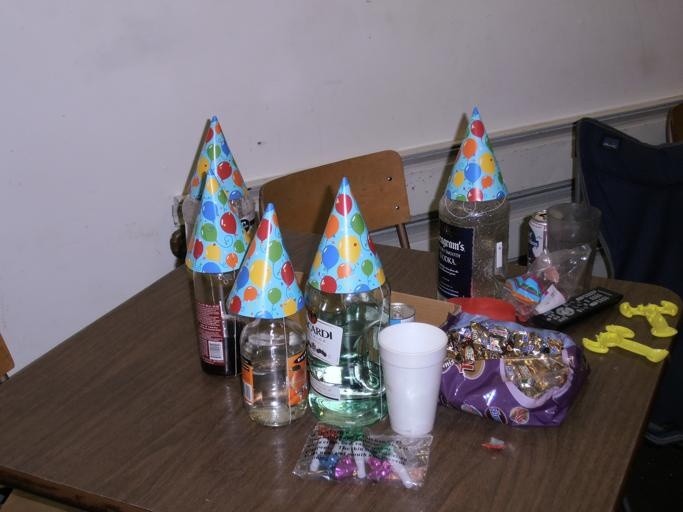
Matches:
[530,286,624,331]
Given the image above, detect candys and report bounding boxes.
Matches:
[439,305,592,430]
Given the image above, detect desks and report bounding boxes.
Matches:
[0,230,680,512]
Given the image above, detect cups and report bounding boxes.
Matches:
[546,202,601,298]
[376,322,448,436]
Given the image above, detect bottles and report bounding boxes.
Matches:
[383,301,417,326]
[528,210,550,267]
[184,165,252,378]
[224,202,309,428]
[181,115,259,240]
[303,175,391,429]
[437,105,509,303]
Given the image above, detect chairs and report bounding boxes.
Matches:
[574,118,683,445]
[666,104,683,143]
[0,335,15,377]
[260,151,409,250]
